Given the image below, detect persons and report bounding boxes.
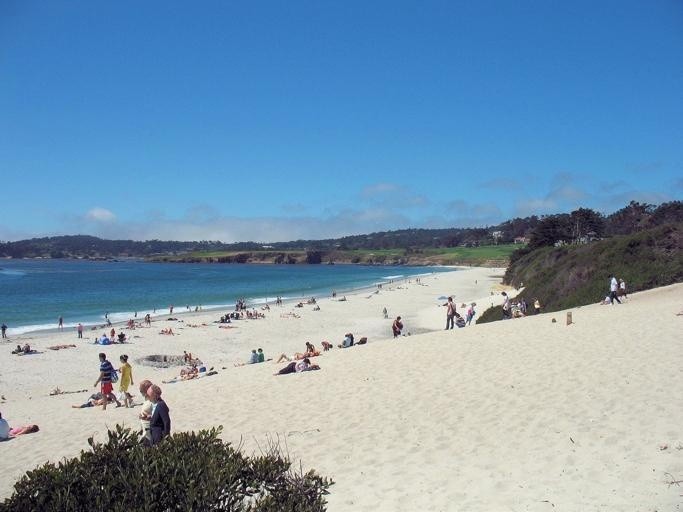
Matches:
[220,292,346,323]
[1,323,8,337]
[6,423,39,437]
[445,297,475,330]
[181,351,200,380]
[145,383,170,445]
[600,293,611,305]
[58,317,63,326]
[153,303,201,335]
[77,323,82,338]
[501,292,540,319]
[236,333,367,375]
[391,316,402,338]
[72,353,133,410]
[15,344,30,353]
[0,412,9,442]
[136,379,153,444]
[98,312,150,345]
[617,278,628,300]
[606,273,622,305]
[378,278,421,288]
